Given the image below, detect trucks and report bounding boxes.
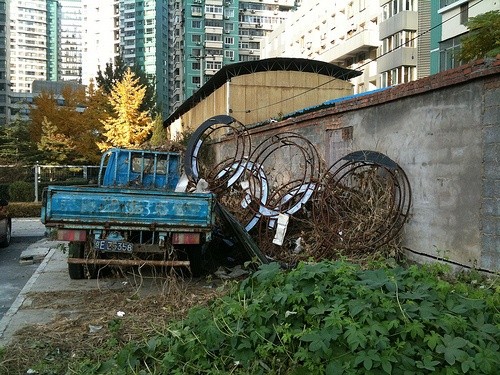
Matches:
[40,145,222,281]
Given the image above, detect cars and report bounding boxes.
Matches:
[0,196,12,248]
